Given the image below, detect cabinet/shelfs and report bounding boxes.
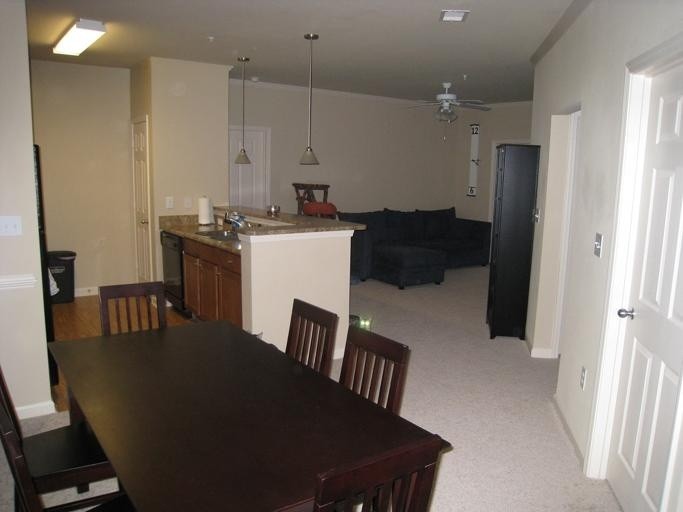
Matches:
[160,232,242,329]
[486,142,541,340]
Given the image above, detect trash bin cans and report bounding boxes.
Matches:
[46,250,76,304]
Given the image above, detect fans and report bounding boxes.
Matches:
[407,84,492,113]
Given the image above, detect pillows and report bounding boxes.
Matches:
[337,208,473,247]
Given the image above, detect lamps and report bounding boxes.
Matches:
[53,18,106,58]
[234,56,251,165]
[435,105,459,125]
[300,33,320,165]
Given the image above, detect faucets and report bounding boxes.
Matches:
[230,214,250,230]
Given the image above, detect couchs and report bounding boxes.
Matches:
[341,218,491,289]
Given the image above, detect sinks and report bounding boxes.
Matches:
[196,229,239,241]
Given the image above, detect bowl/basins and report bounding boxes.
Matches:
[264,205,281,213]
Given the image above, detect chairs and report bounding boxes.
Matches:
[285,298,338,378]
[339,326,411,415]
[313,433,442,511]
[0,367,123,511]
[97,281,167,336]
[292,183,337,219]
